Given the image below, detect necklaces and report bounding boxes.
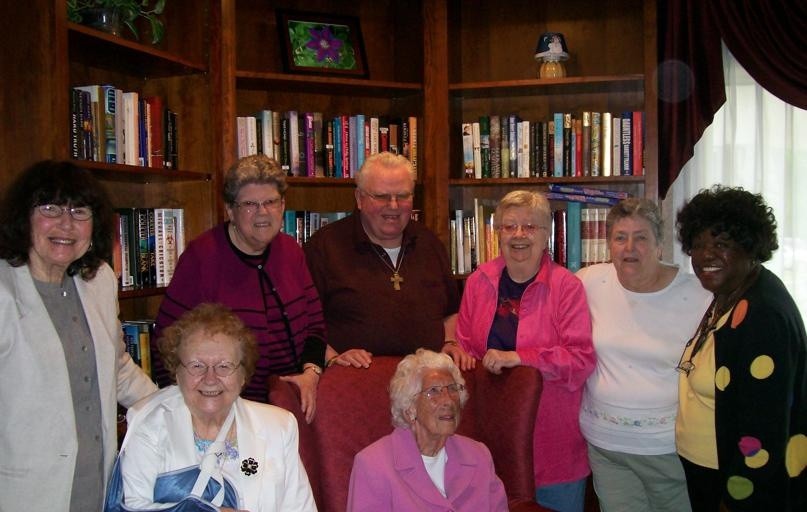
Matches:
[366,236,412,290]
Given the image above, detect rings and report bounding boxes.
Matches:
[489,365,494,368]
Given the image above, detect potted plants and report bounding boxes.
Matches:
[67,1,166,46]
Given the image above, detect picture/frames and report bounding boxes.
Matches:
[274,10,370,81]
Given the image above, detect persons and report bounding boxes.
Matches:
[103,301,320,512]
[344,346,509,512]
[454,191,597,512]
[675,184,807,512]
[151,154,329,426]
[302,150,477,372]
[1,160,161,512]
[570,198,716,512]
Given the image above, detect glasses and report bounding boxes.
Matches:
[36,204,93,220]
[233,199,282,212]
[502,223,546,233]
[675,327,715,374]
[364,190,413,201]
[414,383,462,398]
[177,358,242,377]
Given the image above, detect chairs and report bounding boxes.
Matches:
[266,353,553,511]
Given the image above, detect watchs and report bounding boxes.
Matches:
[303,365,322,375]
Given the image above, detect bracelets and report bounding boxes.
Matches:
[444,340,458,345]
[326,355,337,367]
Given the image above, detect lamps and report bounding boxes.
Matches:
[534,33,572,79]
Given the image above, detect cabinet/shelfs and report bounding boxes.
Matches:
[437,1,667,310]
[228,0,436,248]
[1,0,229,320]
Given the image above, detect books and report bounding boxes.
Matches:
[279,210,351,244]
[107,208,185,289]
[237,110,417,181]
[119,318,159,424]
[545,203,613,274]
[462,111,646,180]
[69,83,180,169]
[450,205,501,275]
